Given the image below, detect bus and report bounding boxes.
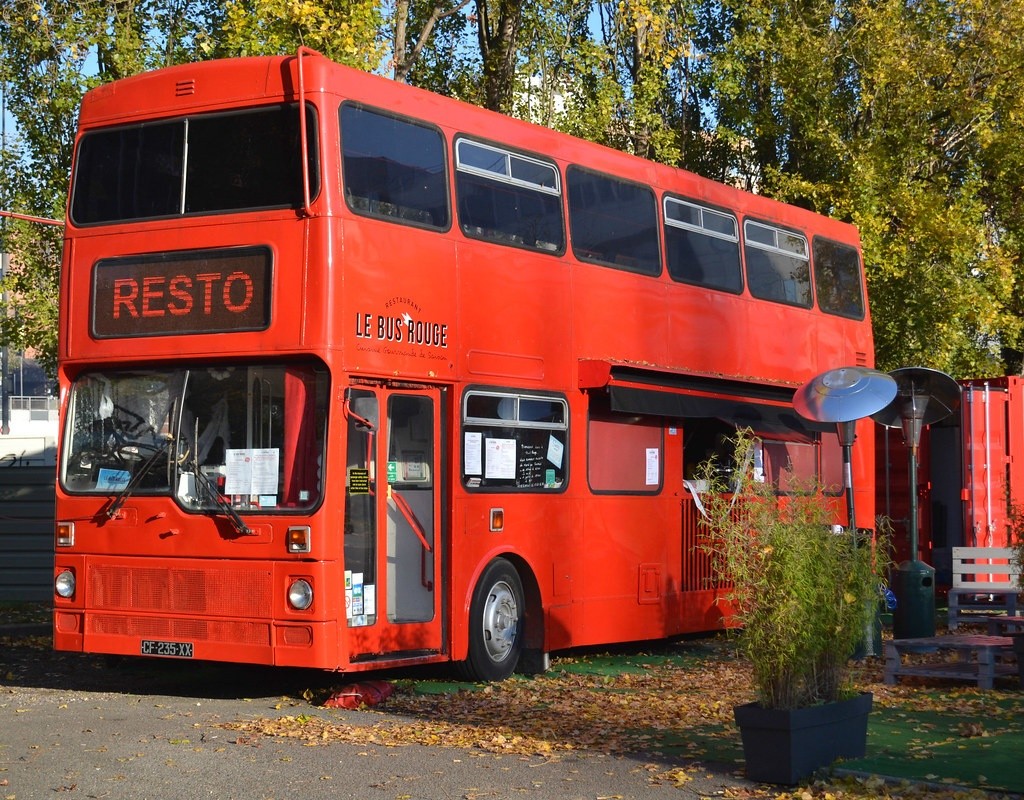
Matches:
[52,43,874,685]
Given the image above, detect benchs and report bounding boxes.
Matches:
[947,547,1024,634]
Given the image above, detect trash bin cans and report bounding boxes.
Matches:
[849,586,883,657]
[892,560,936,639]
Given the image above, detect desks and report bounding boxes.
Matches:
[882,616,1024,689]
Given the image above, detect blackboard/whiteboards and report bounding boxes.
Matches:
[516,443,545,488]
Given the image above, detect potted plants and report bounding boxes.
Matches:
[687,422,899,786]
